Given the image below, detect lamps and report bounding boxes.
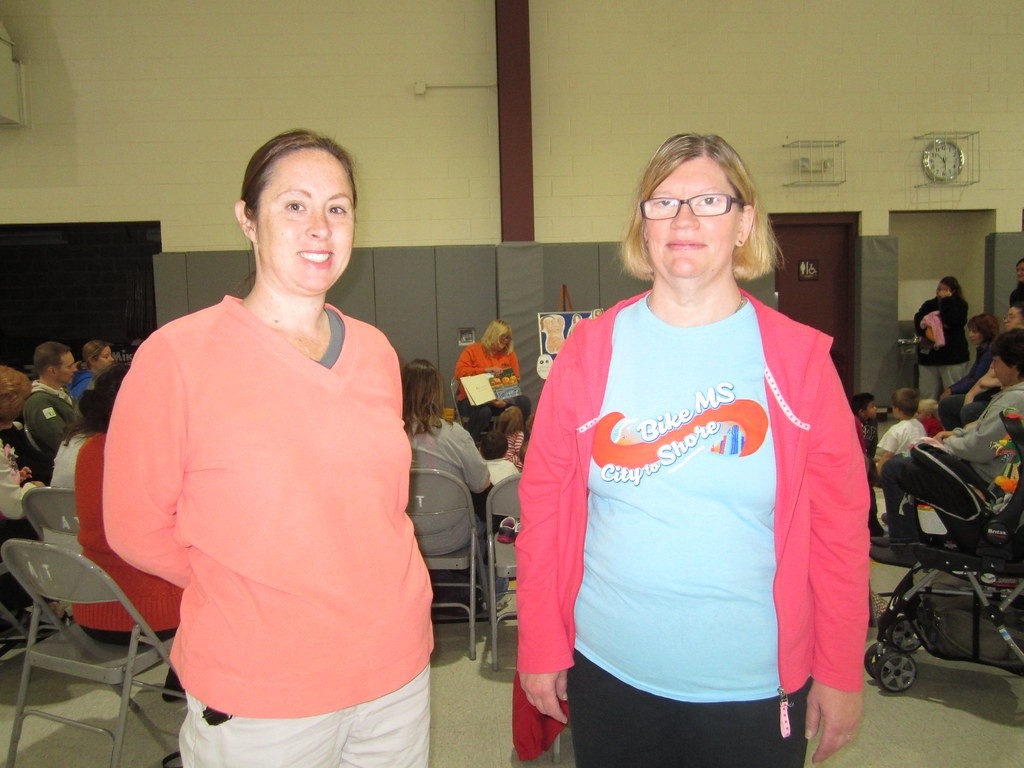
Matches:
[799,157,832,171]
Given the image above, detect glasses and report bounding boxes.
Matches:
[1002,314,1021,321]
[639,193,747,221]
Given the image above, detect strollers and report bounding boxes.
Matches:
[864,405,1024,693]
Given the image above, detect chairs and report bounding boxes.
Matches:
[0,374,522,768]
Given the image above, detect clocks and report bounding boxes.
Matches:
[921,138,965,186]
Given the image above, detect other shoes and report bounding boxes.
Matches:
[871,536,891,548]
[27,614,71,639]
[881,512,890,525]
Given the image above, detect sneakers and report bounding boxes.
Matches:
[498,517,519,544]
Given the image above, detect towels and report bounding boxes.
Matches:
[920,311,945,346]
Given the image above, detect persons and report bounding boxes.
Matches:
[44,363,184,703]
[23,342,81,454]
[481,395,536,472]
[853,387,944,523]
[939,302,1024,431]
[66,341,114,400]
[870,327,1024,569]
[455,320,531,439]
[914,276,970,400]
[0,365,46,520]
[400,358,513,614]
[102,129,433,768]
[514,133,871,768]
[1009,258,1024,306]
